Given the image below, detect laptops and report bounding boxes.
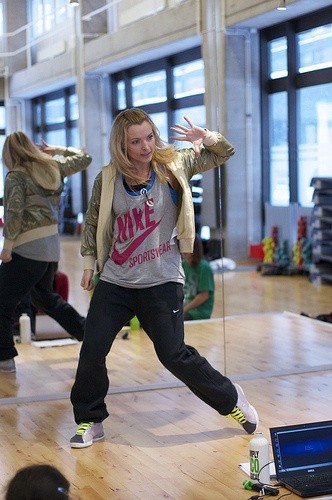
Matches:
[32,315,73,340]
[269,420,332,498]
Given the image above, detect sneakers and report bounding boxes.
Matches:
[0,359,15,373]
[70,422,105,447]
[229,384,258,434]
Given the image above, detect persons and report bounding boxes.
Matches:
[177,231,215,322]
[0,464,70,500]
[70,109,256,449]
[0,130,92,375]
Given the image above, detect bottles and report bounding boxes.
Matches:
[19,312,31,343]
[248,431,270,484]
